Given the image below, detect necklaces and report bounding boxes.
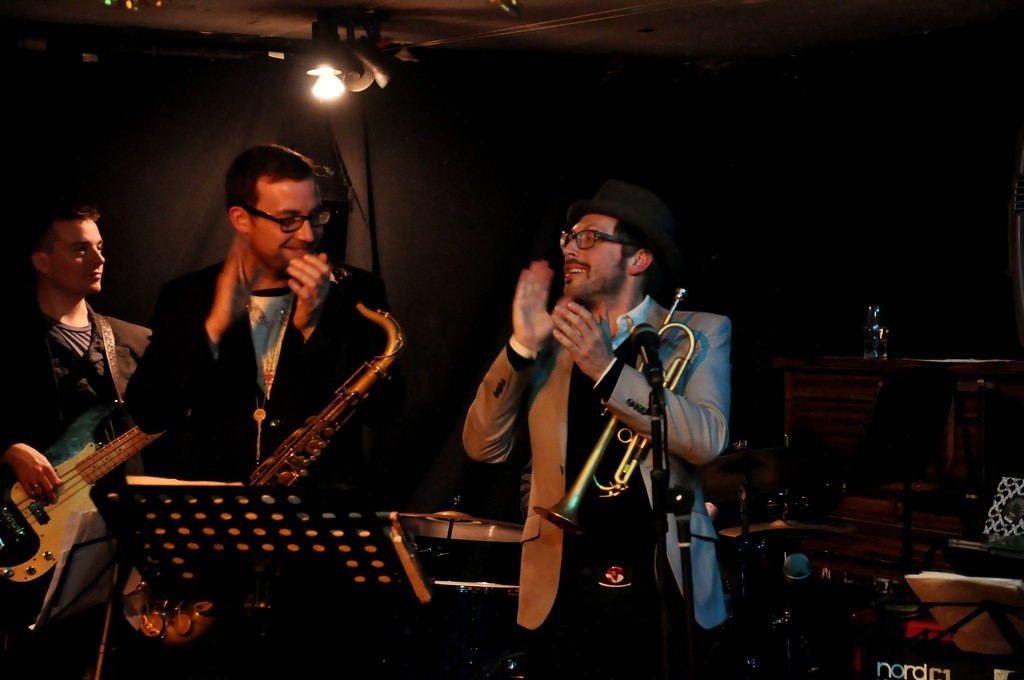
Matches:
[253,299,289,464]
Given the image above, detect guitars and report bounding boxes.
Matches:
[1,426,163,585]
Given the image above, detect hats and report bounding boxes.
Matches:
[567,179,682,278]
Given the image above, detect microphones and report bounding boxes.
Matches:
[631,323,667,406]
[863,304,881,359]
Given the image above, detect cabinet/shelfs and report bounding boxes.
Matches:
[767,357,1024,546]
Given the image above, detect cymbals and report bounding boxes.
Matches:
[391,508,525,547]
[718,520,861,540]
[692,445,825,496]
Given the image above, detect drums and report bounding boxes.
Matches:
[419,575,518,679]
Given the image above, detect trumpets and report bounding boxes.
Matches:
[530,285,699,536]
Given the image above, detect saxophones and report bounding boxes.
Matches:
[117,250,407,646]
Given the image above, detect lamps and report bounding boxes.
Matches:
[349,34,400,90]
[307,19,345,98]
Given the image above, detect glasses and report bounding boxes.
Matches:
[559,229,641,251]
[237,202,334,233]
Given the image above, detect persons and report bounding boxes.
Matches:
[462,175,733,680]
[0,201,154,680]
[124,144,409,680]
